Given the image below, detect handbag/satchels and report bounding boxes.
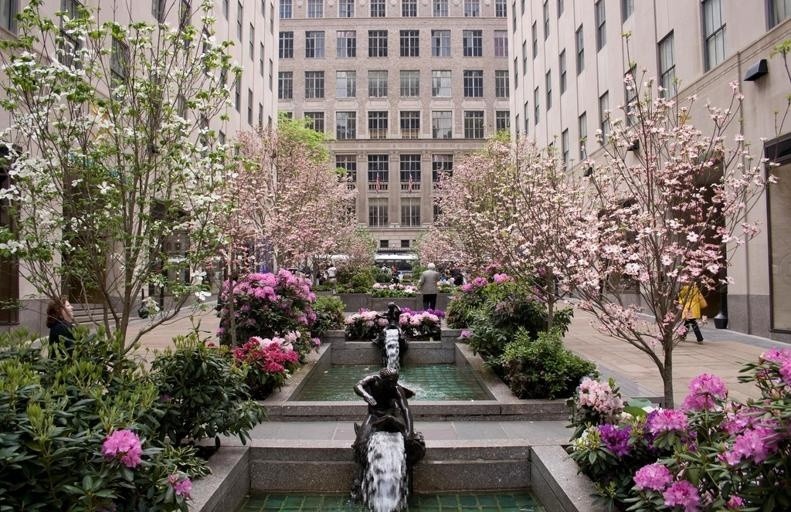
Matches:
[698,290,708,309]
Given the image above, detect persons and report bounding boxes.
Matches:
[677,282,709,346]
[372,301,407,345]
[381,264,387,273]
[353,366,416,448]
[419,262,441,312]
[44,293,83,361]
[300,262,339,291]
